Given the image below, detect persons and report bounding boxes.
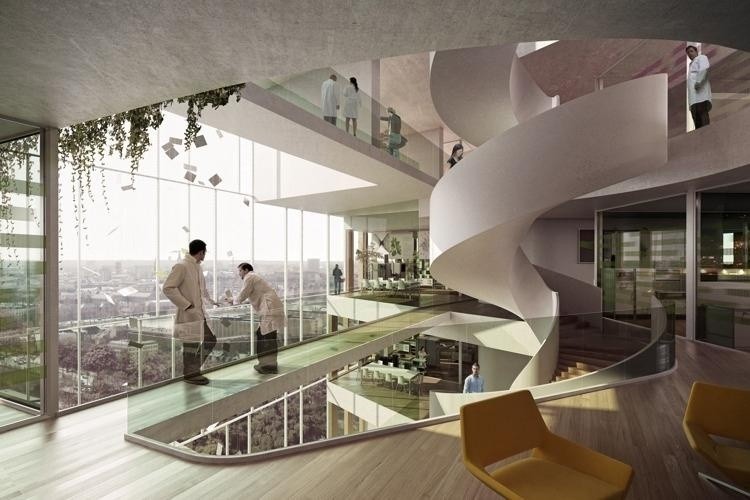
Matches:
[446,143,463,171]
[381,107,402,158]
[320,73,340,126]
[462,361,484,394]
[418,344,427,364]
[225,262,285,374]
[163,239,217,385]
[332,264,343,295]
[343,77,362,137]
[683,44,713,129]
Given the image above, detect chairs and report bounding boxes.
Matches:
[358,276,421,301]
[460,379,750,500]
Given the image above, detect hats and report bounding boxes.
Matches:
[187,239,207,256]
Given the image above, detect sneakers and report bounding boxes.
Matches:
[252,364,279,375]
[183,375,210,385]
